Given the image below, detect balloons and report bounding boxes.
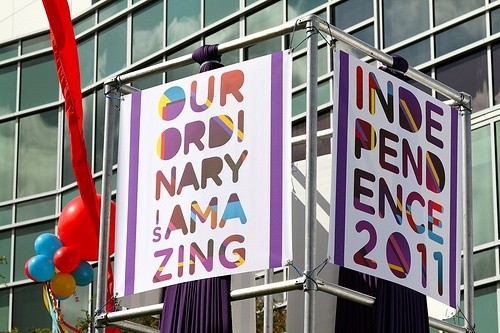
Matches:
[24,193,116,300]
